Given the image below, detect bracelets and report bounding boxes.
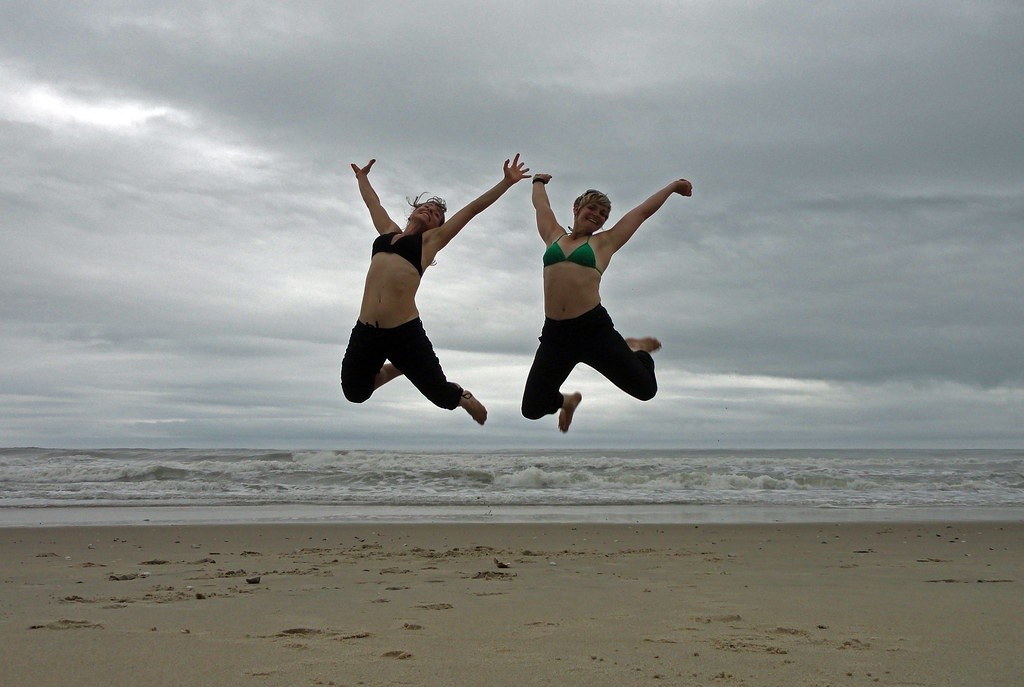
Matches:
[532,178,545,184]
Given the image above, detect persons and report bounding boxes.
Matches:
[341,153,531,425]
[521,174,692,432]
